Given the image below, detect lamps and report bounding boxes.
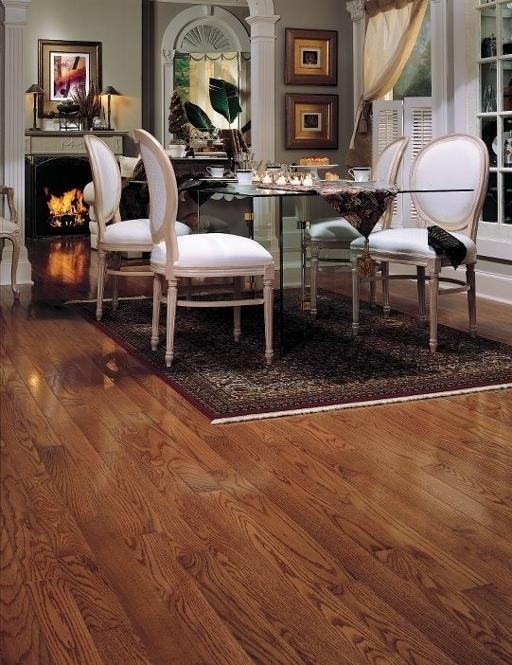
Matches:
[25,84,45,130]
[96,84,123,128]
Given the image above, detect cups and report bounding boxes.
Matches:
[235,167,254,186]
[206,164,225,177]
[347,165,371,181]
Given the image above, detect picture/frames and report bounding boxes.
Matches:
[283,90,339,149]
[37,38,103,119]
[283,25,339,85]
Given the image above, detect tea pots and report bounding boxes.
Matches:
[208,141,228,157]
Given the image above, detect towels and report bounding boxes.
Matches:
[428,225,466,269]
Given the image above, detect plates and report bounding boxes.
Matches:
[343,179,383,183]
[198,176,240,182]
[289,163,339,179]
[318,178,341,184]
[226,183,260,190]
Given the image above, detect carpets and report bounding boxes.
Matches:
[79,283,511,426]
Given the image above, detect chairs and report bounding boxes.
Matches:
[0,185,21,298]
[352,133,491,351]
[305,134,411,319]
[84,132,191,322]
[133,127,276,368]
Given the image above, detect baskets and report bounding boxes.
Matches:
[300,157,329,168]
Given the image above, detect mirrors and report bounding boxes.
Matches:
[160,5,252,156]
